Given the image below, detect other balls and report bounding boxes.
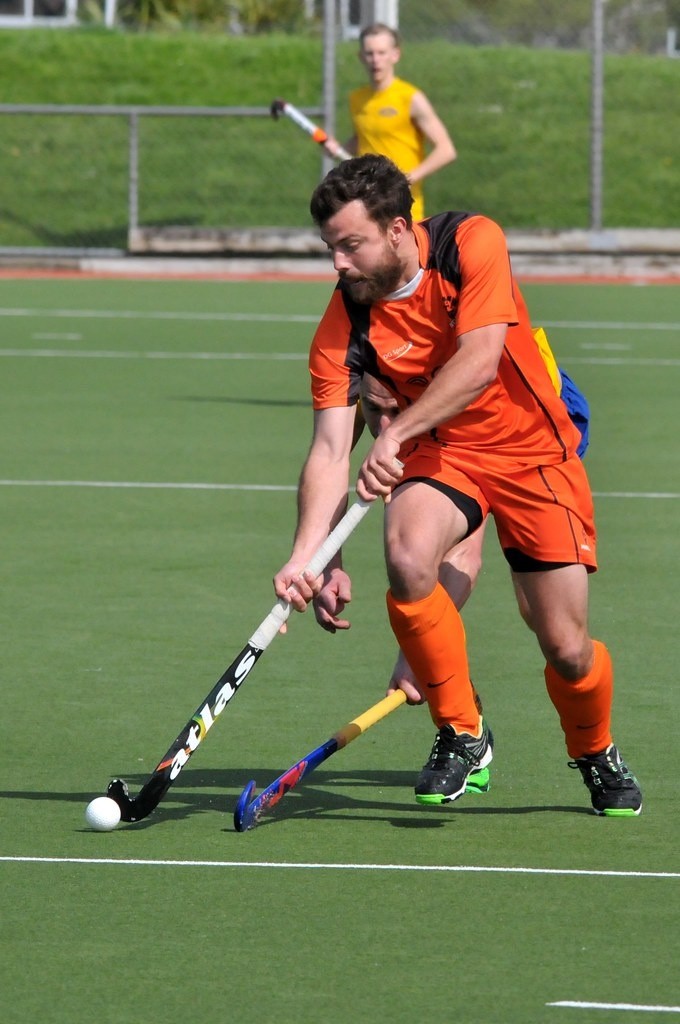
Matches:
[84,796,121,834]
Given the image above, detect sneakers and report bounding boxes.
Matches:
[567,743,643,816]
[416,713,494,806]
[465,767,490,793]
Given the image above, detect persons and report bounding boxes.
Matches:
[323,22,456,223]
[270,154,644,818]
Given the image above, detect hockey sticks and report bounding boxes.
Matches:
[231,687,409,834]
[111,453,406,823]
[268,96,355,162]
